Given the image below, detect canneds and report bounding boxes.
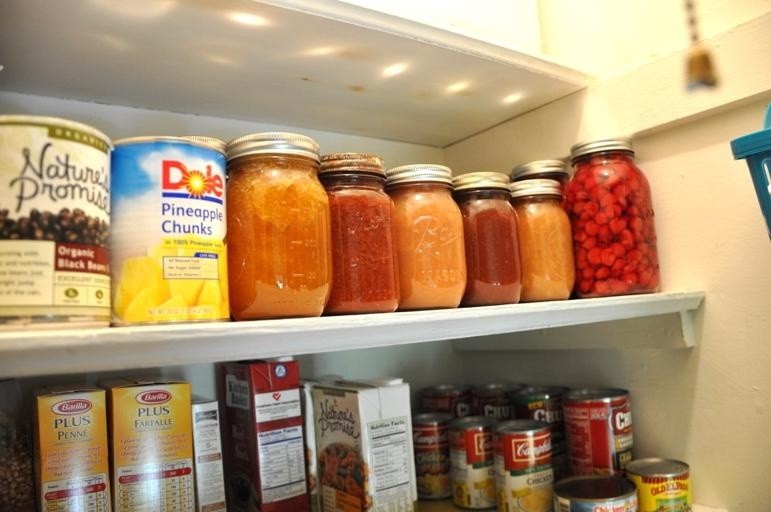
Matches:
[319,151,402,314]
[384,164,468,310]
[509,178,576,303]
[0,115,226,325]
[566,135,663,298]
[512,159,572,185]
[452,170,524,307]
[413,384,694,512]
[225,130,333,320]
